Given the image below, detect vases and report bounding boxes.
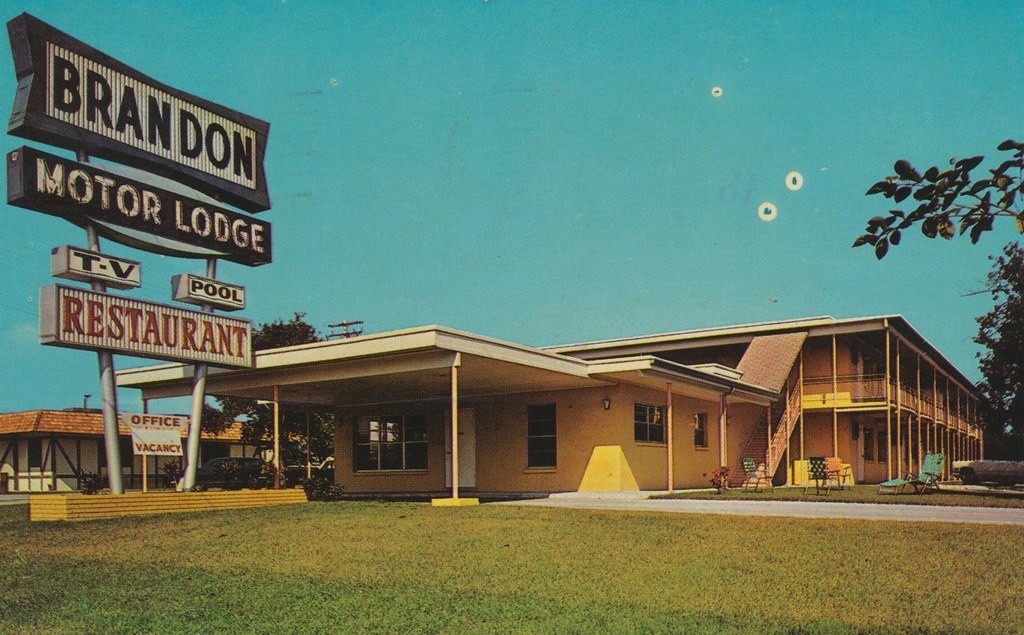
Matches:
[717,488,726,494]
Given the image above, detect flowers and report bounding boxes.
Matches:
[709,466,731,487]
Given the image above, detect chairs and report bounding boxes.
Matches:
[878,453,944,499]
[741,458,773,493]
[803,457,852,496]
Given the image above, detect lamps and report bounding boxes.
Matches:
[602,395,610,409]
